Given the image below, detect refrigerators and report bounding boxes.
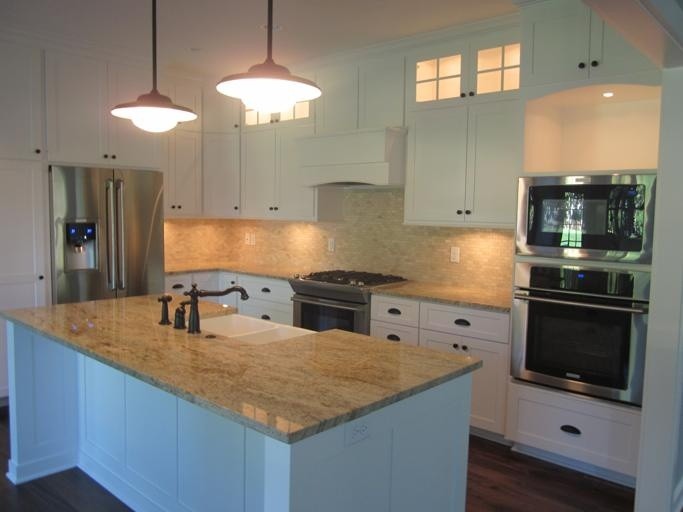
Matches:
[46,164,167,304]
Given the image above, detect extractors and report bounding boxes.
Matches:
[309,180,373,190]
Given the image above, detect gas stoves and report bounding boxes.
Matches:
[289,270,405,287]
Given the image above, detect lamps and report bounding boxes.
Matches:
[216,0,320,116]
[108,1,198,135]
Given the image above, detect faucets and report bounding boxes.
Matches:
[182,282,249,335]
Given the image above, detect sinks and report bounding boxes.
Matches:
[238,326,312,344]
[198,316,276,335]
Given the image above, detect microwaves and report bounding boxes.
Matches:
[519,175,649,264]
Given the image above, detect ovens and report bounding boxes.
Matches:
[291,296,366,336]
[511,263,650,405]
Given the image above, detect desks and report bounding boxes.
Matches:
[0,291,483,512]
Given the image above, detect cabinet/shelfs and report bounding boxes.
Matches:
[368,295,419,352]
[518,1,663,87]
[241,99,313,130]
[45,43,168,171]
[148,271,218,305]
[0,25,44,163]
[168,76,202,130]
[201,132,242,219]
[315,45,408,134]
[202,80,243,130]
[505,379,641,494]
[218,272,296,327]
[165,131,202,219]
[420,303,511,450]
[239,130,343,222]
[0,160,53,407]
[404,96,524,229]
